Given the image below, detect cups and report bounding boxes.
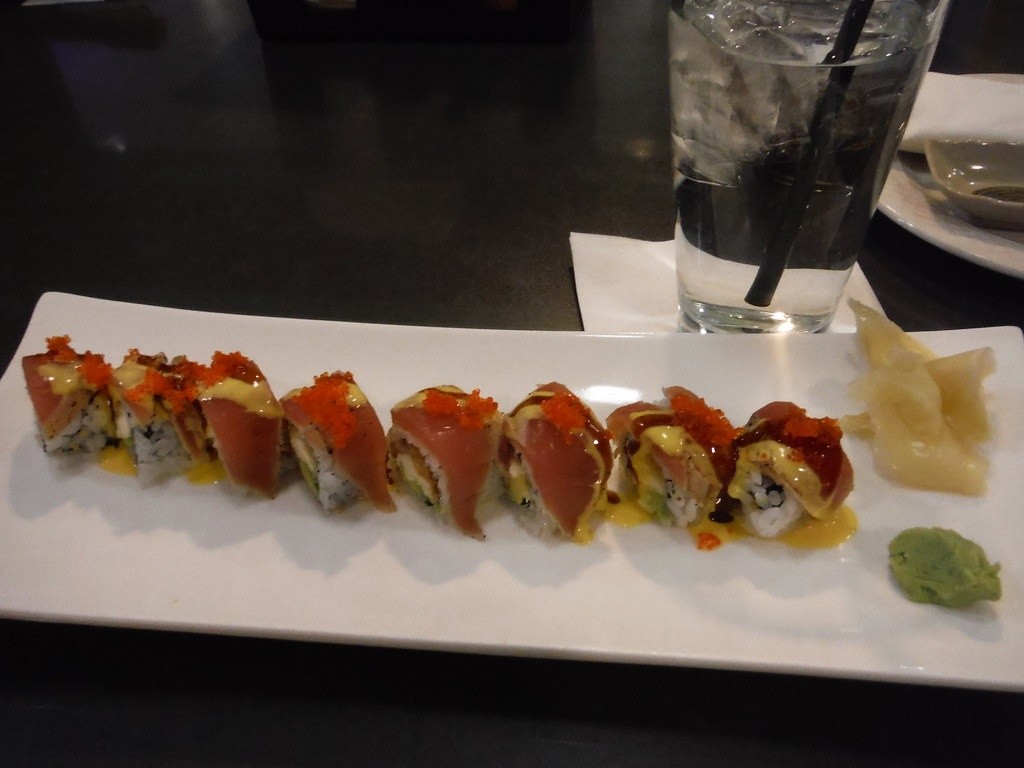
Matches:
[668,0,948,334]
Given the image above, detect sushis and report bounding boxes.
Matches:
[22,333,854,539]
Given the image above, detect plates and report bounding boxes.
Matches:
[877,72,1024,279]
[0,290,1024,690]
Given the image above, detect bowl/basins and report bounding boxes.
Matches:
[924,137,1024,231]
